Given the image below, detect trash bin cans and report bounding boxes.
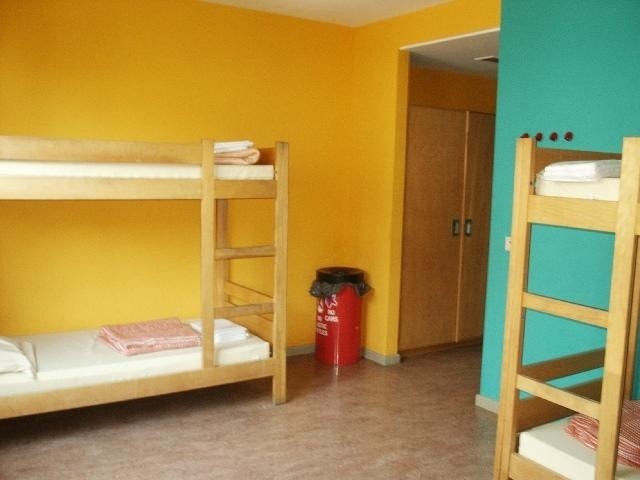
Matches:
[310,267,371,366]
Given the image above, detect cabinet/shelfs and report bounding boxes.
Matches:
[397,107,495,360]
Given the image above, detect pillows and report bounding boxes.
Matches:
[0,335,37,379]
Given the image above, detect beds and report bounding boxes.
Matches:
[494,139,640,480]
[0,139,290,420]
